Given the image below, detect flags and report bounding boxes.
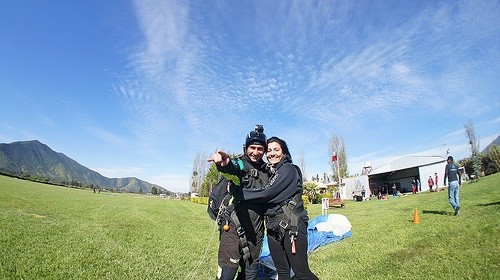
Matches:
[332,152,337,161]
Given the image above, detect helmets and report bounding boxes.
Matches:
[244,131,267,150]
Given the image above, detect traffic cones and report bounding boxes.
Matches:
[412,207,421,225]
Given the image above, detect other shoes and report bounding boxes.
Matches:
[454,206,460,216]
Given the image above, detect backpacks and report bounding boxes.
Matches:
[206,158,244,220]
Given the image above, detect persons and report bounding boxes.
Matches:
[207,124,272,280]
[443,156,461,216]
[227,136,320,280]
[434,172,439,192]
[416,179,421,194]
[411,179,416,194]
[427,175,434,192]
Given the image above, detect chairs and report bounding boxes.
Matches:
[321,198,329,216]
